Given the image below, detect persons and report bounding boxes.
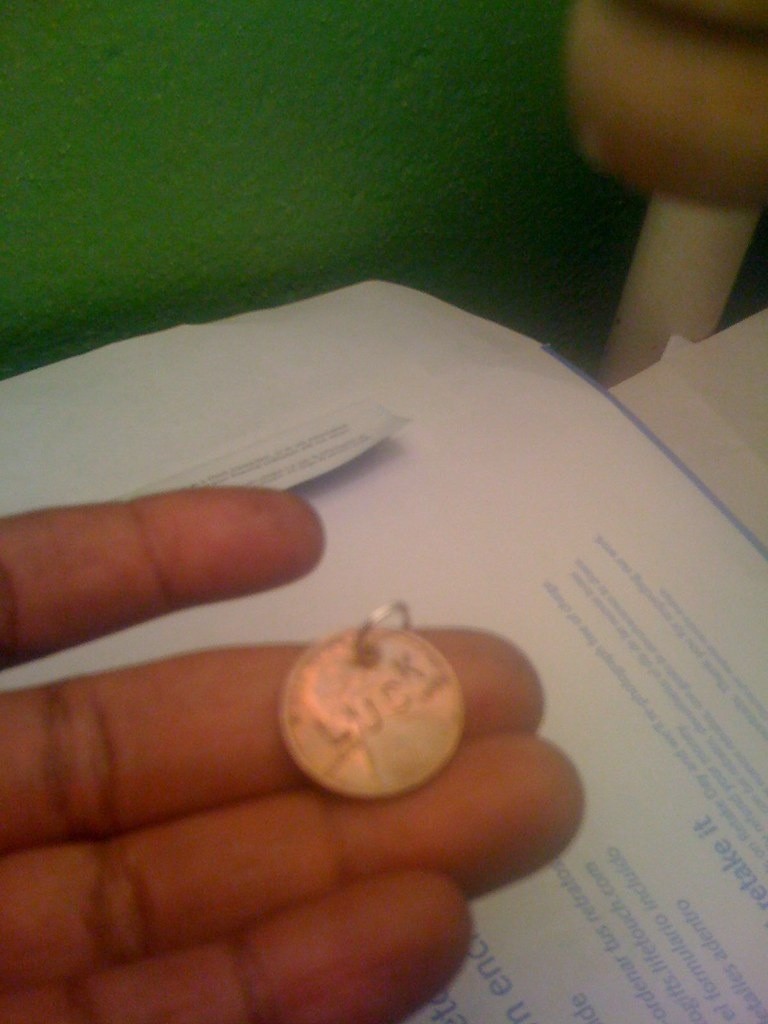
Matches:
[0,486,586,1024]
